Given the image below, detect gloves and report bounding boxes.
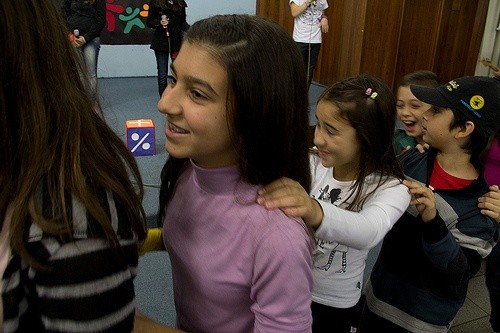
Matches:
[135,227,162,257]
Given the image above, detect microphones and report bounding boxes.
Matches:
[161,15,169,36]
[74,29,79,38]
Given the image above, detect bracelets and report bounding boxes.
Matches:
[322,16,328,20]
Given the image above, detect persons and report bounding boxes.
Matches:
[0,0,149,333]
[65,0,500,333]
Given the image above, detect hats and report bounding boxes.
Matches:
[410,76,500,139]
